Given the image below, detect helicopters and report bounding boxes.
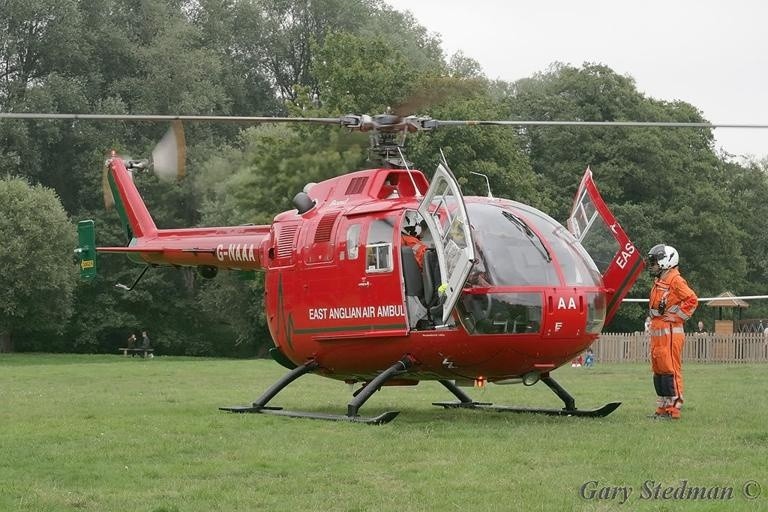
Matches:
[0,114,767,425]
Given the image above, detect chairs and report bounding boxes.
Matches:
[380,246,446,327]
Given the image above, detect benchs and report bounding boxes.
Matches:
[119,347,154,359]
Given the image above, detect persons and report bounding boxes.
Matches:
[391,208,429,272]
[584,349,594,368]
[644,243,698,421]
[141,331,153,359]
[764,327,768,358]
[571,355,583,367]
[643,314,653,335]
[127,334,144,359]
[696,320,708,358]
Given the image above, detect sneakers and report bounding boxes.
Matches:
[652,408,680,418]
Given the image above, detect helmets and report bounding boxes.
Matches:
[648,244,679,278]
